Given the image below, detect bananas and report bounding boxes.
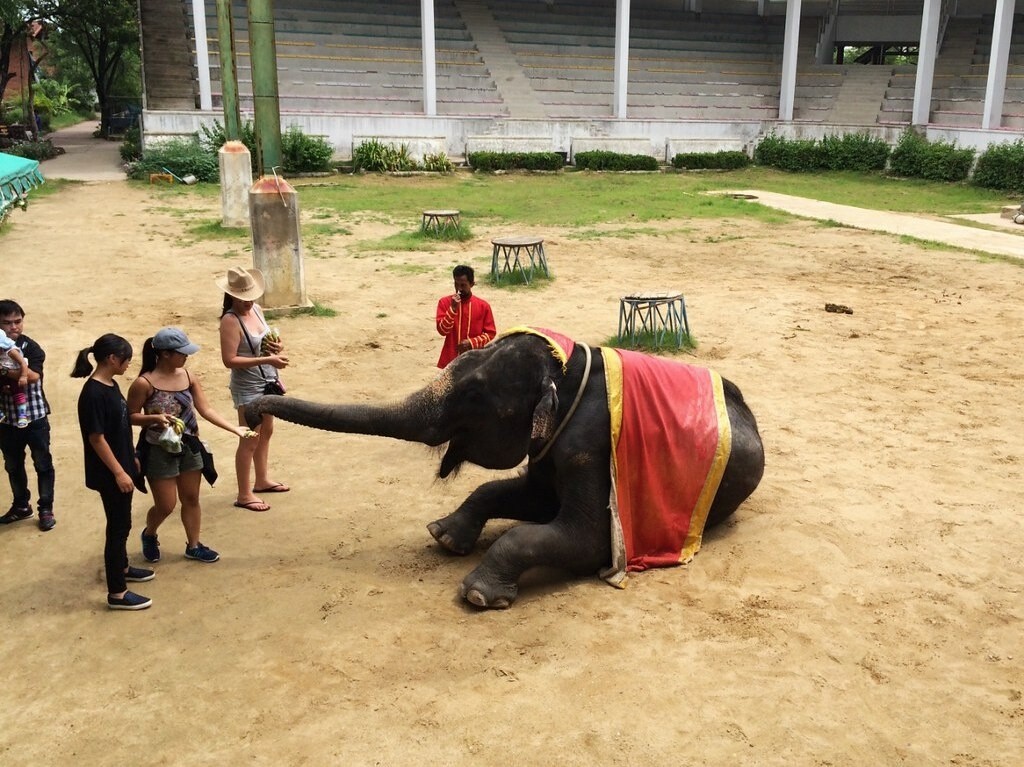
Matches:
[168,416,186,434]
[261,332,277,352]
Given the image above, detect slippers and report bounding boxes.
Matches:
[253,481,290,492]
[234,500,270,511]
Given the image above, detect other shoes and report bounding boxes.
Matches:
[0,411,6,423]
[108,591,152,609]
[17,416,28,428]
[125,566,155,582]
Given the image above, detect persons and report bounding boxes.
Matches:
[69,334,156,610]
[215,267,289,511]
[436,266,496,369]
[127,327,260,563]
[0,300,56,530]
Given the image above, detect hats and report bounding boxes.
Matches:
[215,265,266,301]
[0,328,15,350]
[153,328,199,355]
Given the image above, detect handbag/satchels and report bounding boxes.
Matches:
[264,380,285,395]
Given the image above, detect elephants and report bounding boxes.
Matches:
[246,328,766,607]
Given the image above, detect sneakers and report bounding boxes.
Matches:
[0,504,34,523]
[142,527,161,562]
[38,506,56,531]
[184,541,220,561]
